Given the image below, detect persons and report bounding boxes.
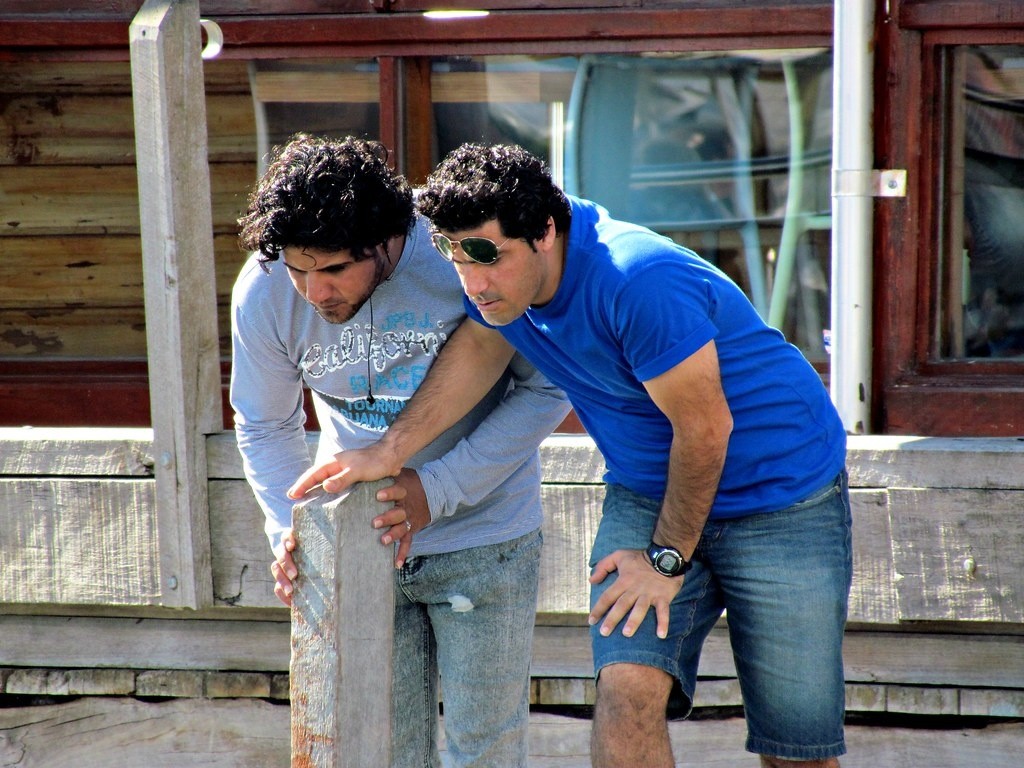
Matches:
[229,133,576,768]
[285,145,854,768]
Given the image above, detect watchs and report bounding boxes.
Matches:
[648,542,694,577]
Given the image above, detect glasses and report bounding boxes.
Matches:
[430,233,511,265]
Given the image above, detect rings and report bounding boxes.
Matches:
[404,520,412,533]
